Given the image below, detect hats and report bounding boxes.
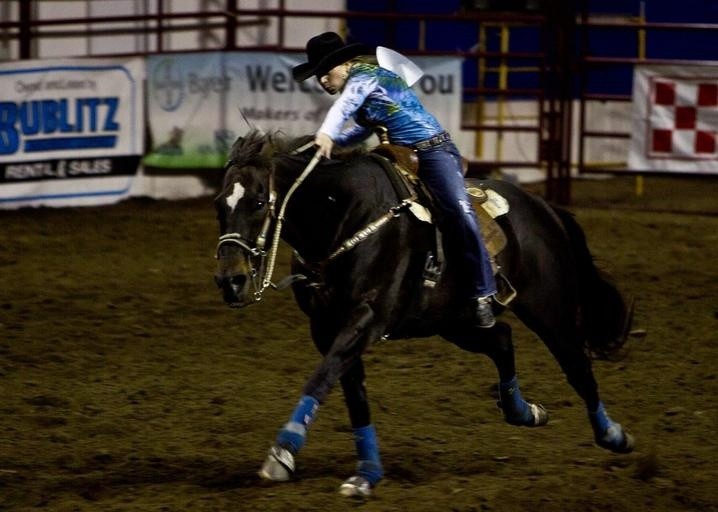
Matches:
[292,32,368,83]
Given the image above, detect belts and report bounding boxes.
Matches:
[408,131,451,151]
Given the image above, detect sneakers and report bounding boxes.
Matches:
[472,296,496,328]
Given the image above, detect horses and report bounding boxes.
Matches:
[213,123,638,500]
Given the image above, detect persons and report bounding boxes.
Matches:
[290,31,500,332]
